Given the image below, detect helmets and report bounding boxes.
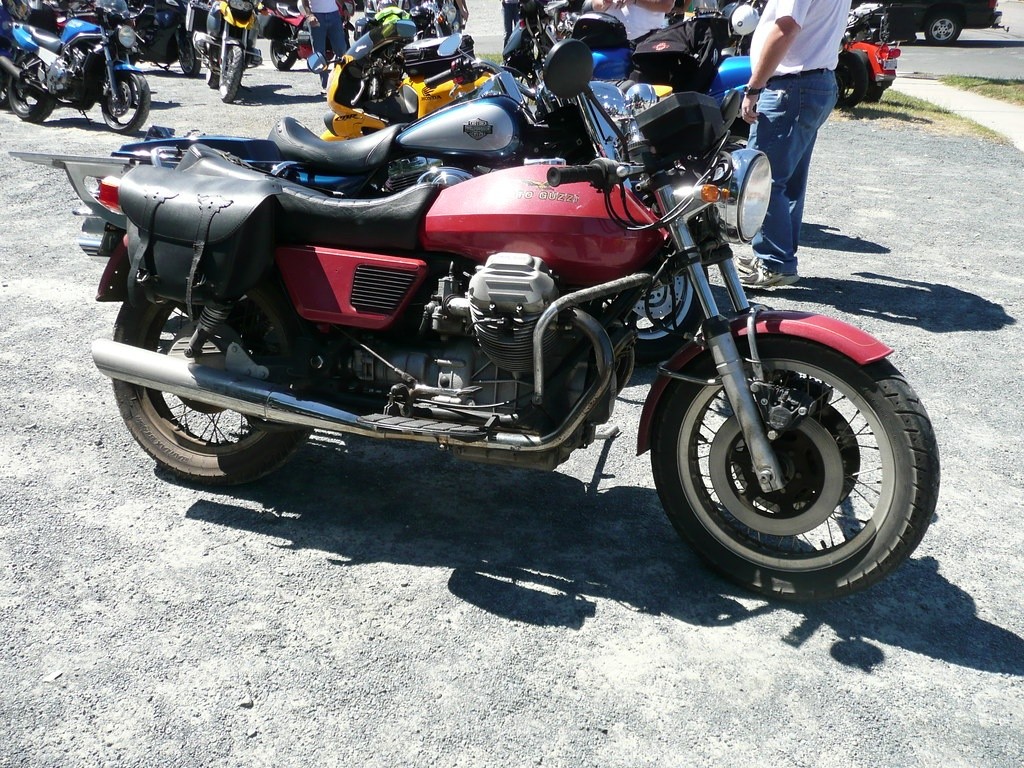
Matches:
[731,3,760,36]
[411,7,431,29]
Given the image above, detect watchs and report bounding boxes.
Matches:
[743,84,765,96]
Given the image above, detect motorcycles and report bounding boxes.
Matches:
[5,6,942,606]
[0,1,903,364]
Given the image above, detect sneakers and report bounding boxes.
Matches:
[734,255,761,274]
[740,266,798,289]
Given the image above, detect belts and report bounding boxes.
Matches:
[768,68,828,81]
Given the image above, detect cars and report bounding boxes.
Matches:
[856,0,1004,47]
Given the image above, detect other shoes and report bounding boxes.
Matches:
[321,89,327,96]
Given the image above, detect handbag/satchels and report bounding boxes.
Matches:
[634,18,734,95]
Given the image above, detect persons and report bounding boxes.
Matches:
[449,0,468,35]
[502,0,521,50]
[296,0,350,95]
[734,0,855,286]
[592,0,720,45]
